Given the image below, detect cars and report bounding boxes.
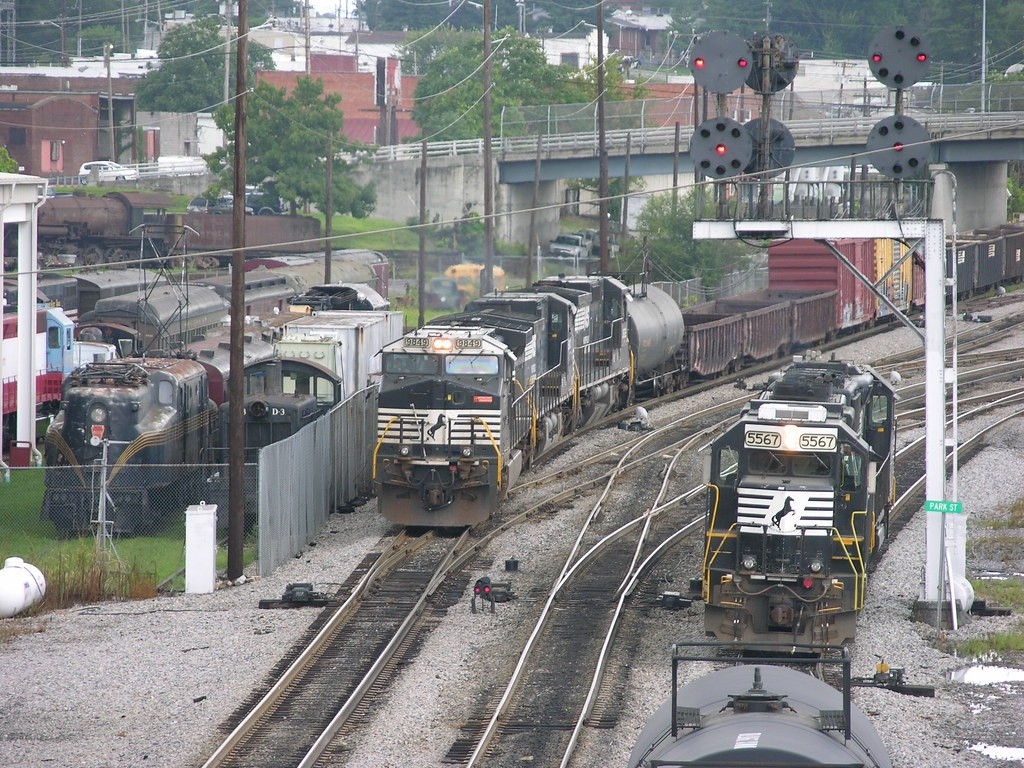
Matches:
[78,160,138,186]
[548,233,593,265]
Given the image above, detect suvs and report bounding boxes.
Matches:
[186,196,255,217]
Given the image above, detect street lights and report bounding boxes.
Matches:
[206,12,232,149]
[39,19,64,57]
[132,19,163,45]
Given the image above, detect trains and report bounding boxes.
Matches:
[371,218,1024,529]
[687,350,901,655]
[3,189,402,542]
[624,638,895,768]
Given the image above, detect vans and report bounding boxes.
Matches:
[571,228,611,253]
